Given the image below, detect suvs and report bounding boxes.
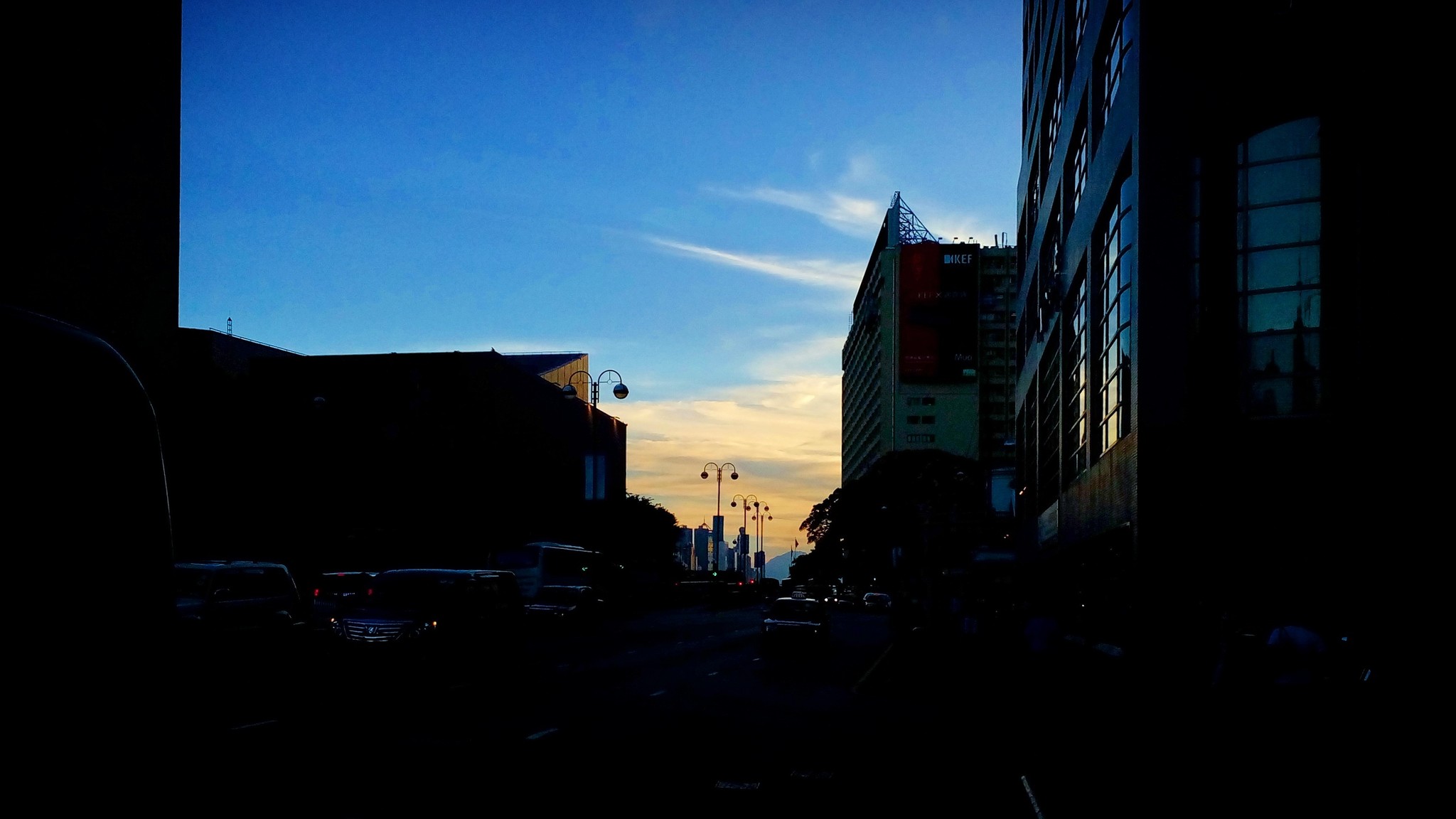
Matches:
[306,572,376,615]
[169,557,297,628]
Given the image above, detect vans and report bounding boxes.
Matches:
[343,570,520,650]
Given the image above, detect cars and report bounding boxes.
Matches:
[517,540,894,647]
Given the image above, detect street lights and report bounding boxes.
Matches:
[562,371,626,533]
[701,461,771,585]
